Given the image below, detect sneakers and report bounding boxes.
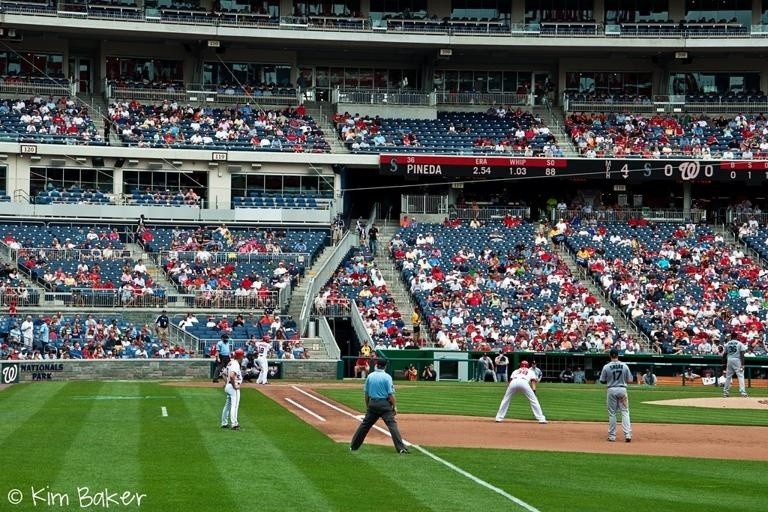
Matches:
[221,424,229,430]
[741,392,748,397]
[625,436,631,442]
[724,389,730,395]
[400,449,411,455]
[231,425,240,430]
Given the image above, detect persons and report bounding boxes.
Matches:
[351,358,410,453]
[221,352,244,430]
[495,360,548,424]
[599,348,633,442]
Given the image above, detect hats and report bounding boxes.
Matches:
[377,359,389,366]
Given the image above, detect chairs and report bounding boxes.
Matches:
[0,0,768,387]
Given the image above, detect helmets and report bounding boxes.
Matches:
[262,335,272,342]
[221,334,229,343]
[235,348,244,360]
[520,360,529,368]
